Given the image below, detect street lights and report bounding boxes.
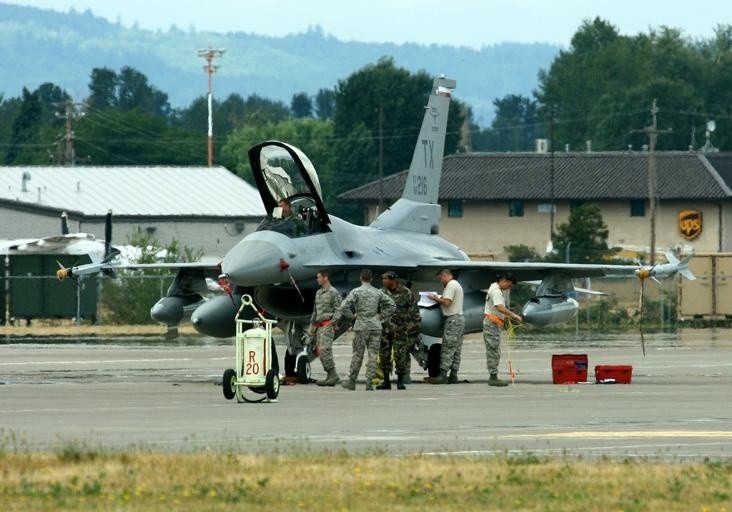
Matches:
[198,43,229,167]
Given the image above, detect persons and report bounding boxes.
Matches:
[339,268,397,391]
[375,270,421,390]
[428,268,465,384]
[308,269,342,387]
[272,198,304,236]
[482,272,521,386]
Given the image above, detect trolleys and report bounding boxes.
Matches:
[219,290,284,405]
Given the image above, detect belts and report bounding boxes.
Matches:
[484,314,504,326]
[314,320,333,328]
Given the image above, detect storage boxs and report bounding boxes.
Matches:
[595,365,632,384]
[552,354,588,384]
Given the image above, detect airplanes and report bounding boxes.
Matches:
[50,73,706,386]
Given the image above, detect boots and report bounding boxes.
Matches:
[316,368,510,391]
